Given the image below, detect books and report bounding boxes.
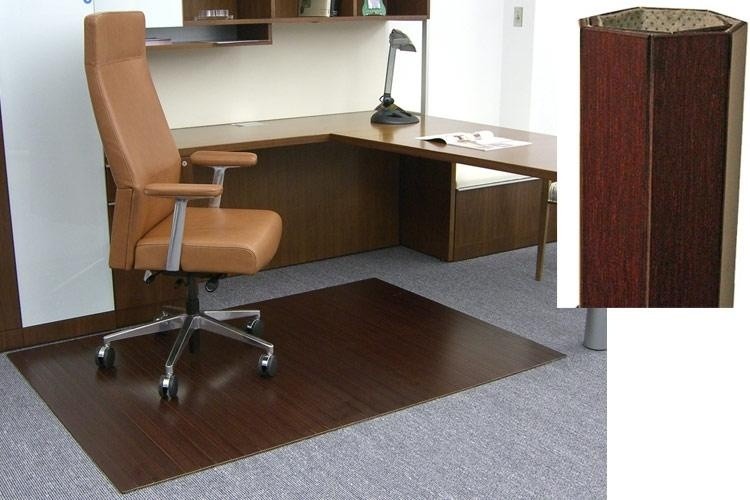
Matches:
[415,130,535,152]
[145,36,176,48]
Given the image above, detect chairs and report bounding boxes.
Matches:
[83,9,285,402]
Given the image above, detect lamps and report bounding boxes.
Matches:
[370,26,421,126]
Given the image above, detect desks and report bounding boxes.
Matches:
[103,110,558,331]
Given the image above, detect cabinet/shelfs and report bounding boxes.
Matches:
[145,0,431,52]
